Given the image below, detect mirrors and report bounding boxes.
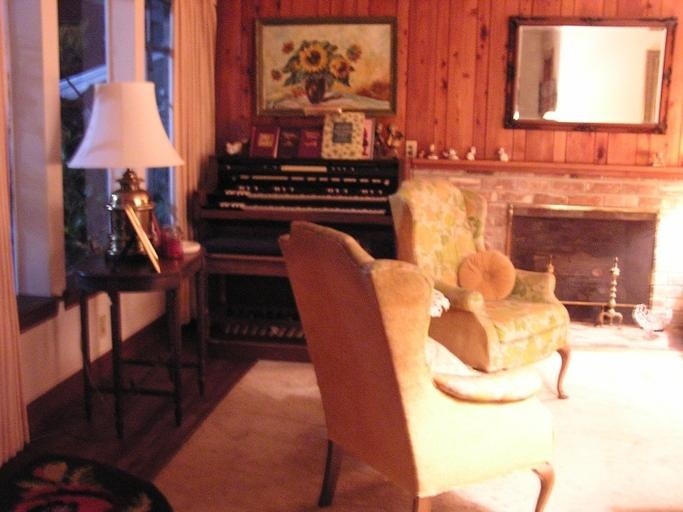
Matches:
[501,14,680,135]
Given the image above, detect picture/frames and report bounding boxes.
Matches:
[252,15,400,130]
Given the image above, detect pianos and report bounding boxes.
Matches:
[189,147,413,361]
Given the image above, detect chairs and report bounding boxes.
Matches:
[391,177,572,401]
[277,221,559,510]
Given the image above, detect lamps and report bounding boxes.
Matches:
[63,79,185,257]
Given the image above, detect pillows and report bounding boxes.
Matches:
[427,334,474,374]
[458,249,516,300]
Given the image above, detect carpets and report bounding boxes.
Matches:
[137,356,683,510]
[0,450,170,510]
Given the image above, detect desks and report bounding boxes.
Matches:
[78,241,209,431]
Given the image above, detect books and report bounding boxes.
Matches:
[248,111,376,160]
